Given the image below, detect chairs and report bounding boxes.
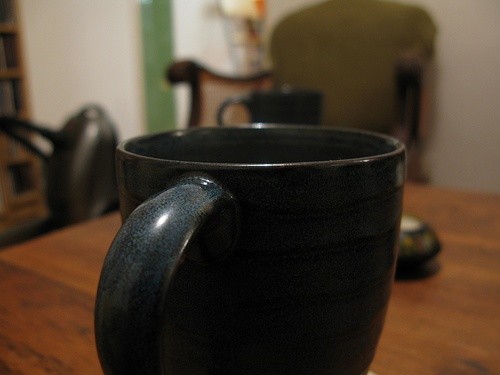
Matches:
[162,0,438,182]
[0,105,121,246]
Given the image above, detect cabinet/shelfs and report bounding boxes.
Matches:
[0,0,50,221]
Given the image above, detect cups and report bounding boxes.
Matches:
[88,122,406,374]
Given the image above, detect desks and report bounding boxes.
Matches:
[1,180,497,372]
